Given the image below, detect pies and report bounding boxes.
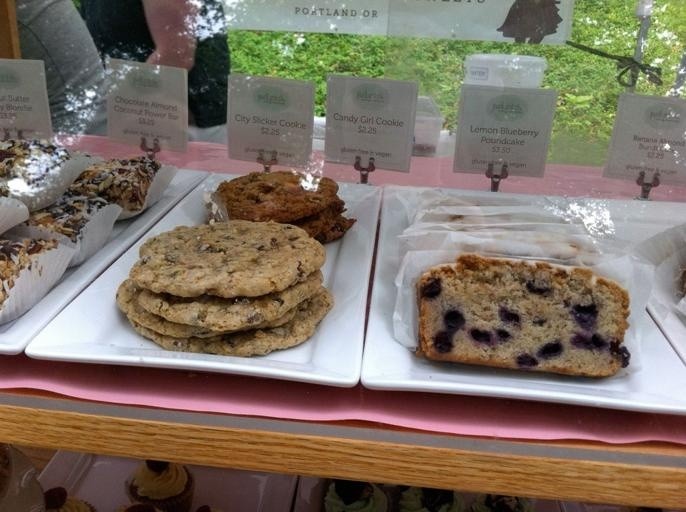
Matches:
[115,170,357,357]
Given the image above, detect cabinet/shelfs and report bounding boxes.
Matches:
[1,1,685,512]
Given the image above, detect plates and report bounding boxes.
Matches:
[358,183,685,416]
[1,150,216,356]
[24,171,384,390]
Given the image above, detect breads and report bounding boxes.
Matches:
[415,255,630,379]
[1,139,160,310]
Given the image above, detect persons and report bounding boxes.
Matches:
[73,0,232,128]
[11,0,110,136]
